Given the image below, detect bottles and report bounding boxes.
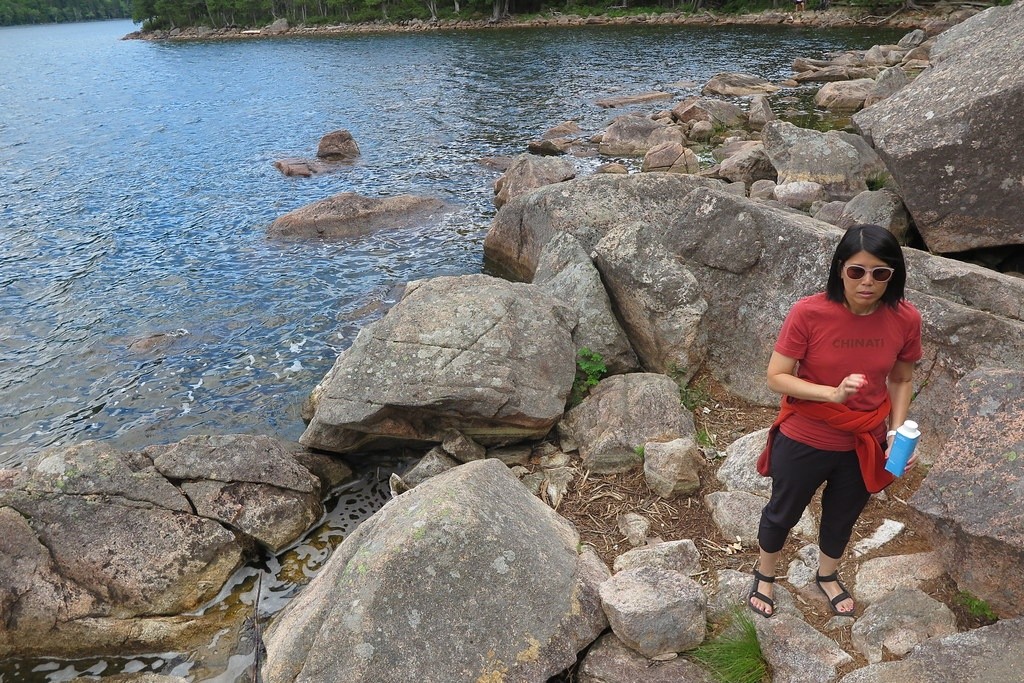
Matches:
[884,420,922,478]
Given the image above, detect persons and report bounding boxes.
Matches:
[819,0,831,11]
[793,0,805,12]
[748,224,922,618]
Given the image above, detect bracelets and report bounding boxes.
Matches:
[886,431,897,440]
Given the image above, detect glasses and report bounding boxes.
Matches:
[844,265,895,282]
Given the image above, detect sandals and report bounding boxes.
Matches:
[747,569,774,618]
[816,567,855,617]
